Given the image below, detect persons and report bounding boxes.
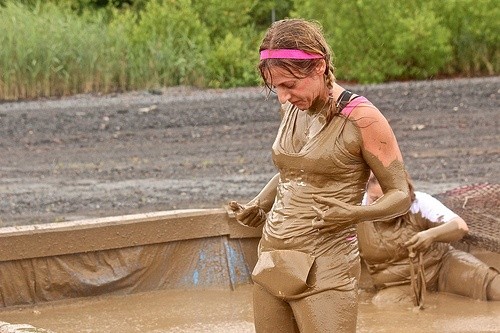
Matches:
[346,164,500,314]
[231,19,411,332]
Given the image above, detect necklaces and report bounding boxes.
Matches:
[296,88,332,137]
